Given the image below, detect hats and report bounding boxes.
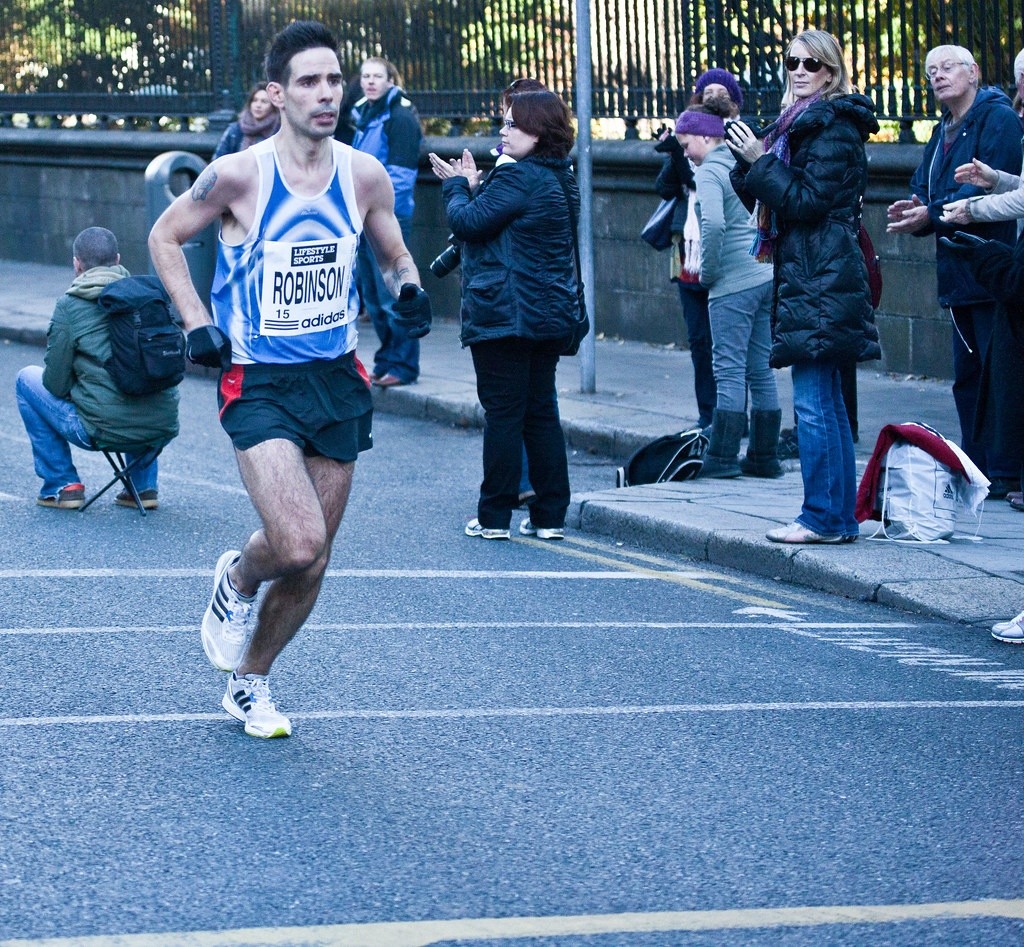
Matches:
[695,67,743,112]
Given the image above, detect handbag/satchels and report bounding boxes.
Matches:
[639,196,682,252]
[561,282,590,356]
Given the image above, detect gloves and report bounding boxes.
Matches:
[651,123,674,145]
[722,121,759,174]
[388,283,433,339]
[186,325,232,372]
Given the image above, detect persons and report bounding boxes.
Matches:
[16,228,179,507]
[654,69,751,437]
[724,29,882,544]
[211,80,282,161]
[777,86,881,460]
[428,79,592,541]
[147,21,433,739]
[886,46,1024,510]
[335,61,402,146]
[940,49,1024,642]
[675,95,783,480]
[349,56,424,388]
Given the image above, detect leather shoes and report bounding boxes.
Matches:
[366,372,377,383]
[372,373,403,386]
[1009,497,1024,512]
[1005,491,1024,502]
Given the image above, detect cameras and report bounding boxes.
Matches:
[429,232,464,279]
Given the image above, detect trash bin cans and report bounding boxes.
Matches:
[142,149,222,328]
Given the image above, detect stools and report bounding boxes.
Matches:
[79,436,165,516]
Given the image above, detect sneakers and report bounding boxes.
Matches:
[221,669,292,739]
[465,517,511,540]
[766,520,858,544]
[519,517,565,540]
[991,610,1024,643]
[200,550,259,672]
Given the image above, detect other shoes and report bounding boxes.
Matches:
[113,487,158,509]
[35,482,86,509]
[987,475,1022,500]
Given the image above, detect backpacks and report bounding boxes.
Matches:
[97,274,187,397]
[866,423,983,546]
[615,428,710,488]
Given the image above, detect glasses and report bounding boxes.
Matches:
[785,56,825,73]
[924,61,971,80]
[503,119,518,129]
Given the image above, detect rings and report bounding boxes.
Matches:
[738,144,743,147]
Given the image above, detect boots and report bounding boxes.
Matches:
[740,408,785,480]
[695,408,747,479]
